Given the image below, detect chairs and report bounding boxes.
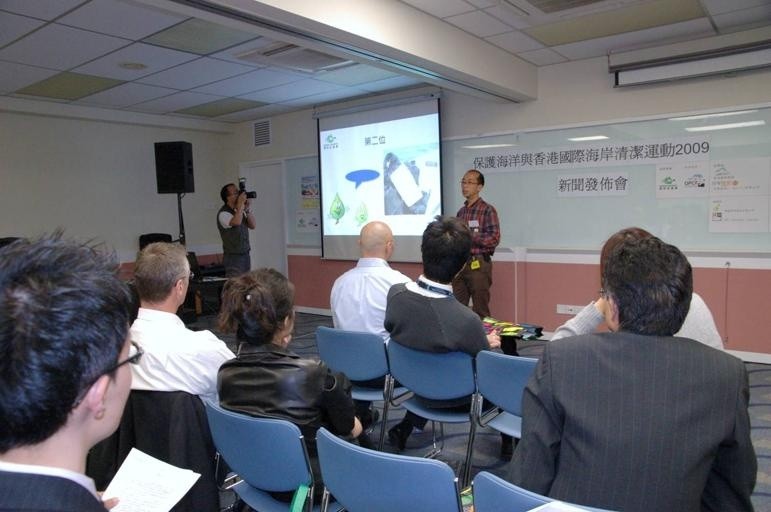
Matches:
[82,327,622,512]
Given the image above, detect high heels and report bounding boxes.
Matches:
[387,420,415,452]
[499,440,515,463]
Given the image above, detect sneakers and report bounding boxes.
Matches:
[355,408,380,431]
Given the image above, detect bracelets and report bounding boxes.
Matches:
[244,208,253,213]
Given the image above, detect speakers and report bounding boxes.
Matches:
[154,141,194,193]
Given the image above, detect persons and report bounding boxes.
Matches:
[216,267,377,506]
[129,242,237,406]
[452,169,501,320]
[330,220,428,449]
[217,183,256,278]
[0,226,145,512]
[550,227,723,350]
[384,214,520,462]
[506,236,758,512]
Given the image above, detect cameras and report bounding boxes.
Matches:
[238,178,256,198]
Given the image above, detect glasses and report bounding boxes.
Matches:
[69,337,147,412]
[458,181,482,186]
[173,270,196,289]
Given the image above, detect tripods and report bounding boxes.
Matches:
[177,193,219,317]
